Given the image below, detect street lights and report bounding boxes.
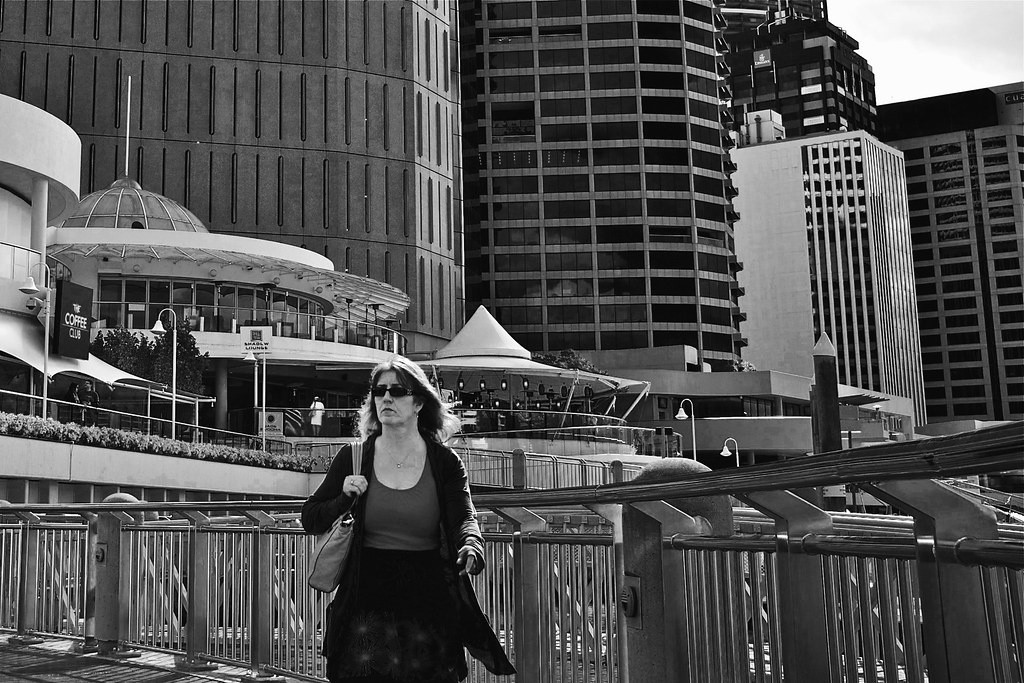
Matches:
[243,340,266,453]
[720,437,740,468]
[18,262,51,421]
[151,308,177,440]
[675,398,697,462]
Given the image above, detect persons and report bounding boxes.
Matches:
[350,480,355,486]
[379,433,423,469]
[78,378,100,427]
[66,381,85,426]
[448,386,619,438]
[310,396,326,436]
[299,362,519,682]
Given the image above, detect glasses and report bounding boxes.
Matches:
[372,385,413,398]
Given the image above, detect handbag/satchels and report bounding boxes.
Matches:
[308,401,317,417]
[307,442,363,594]
